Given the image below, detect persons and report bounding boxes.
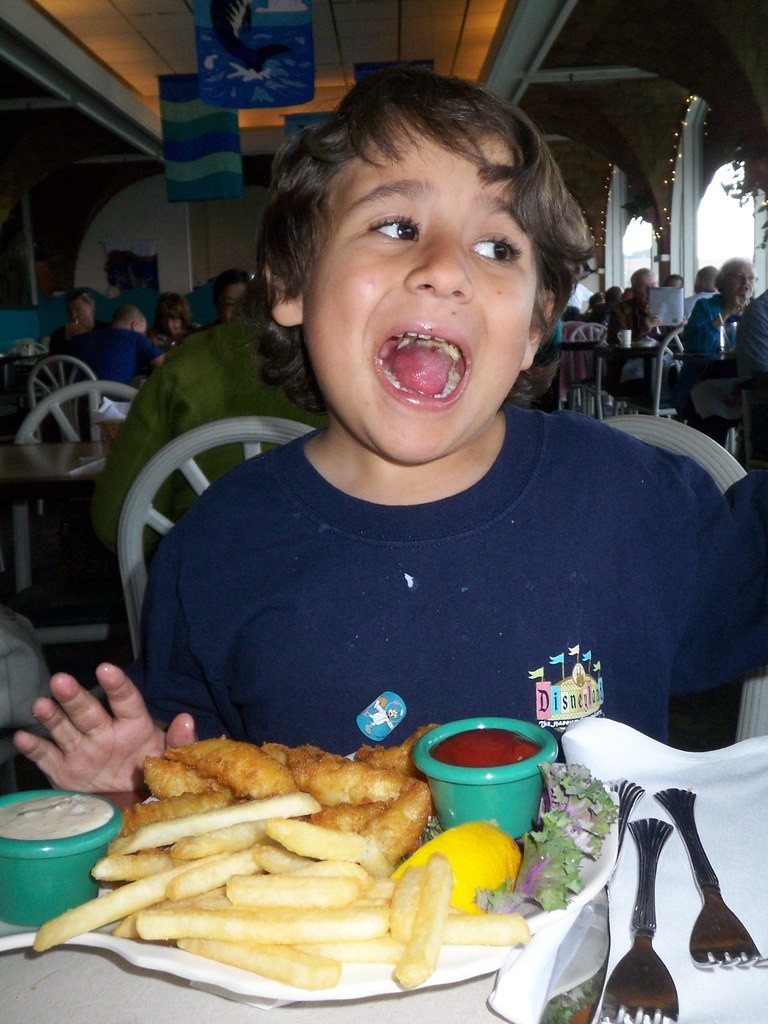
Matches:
[15,62,768,793]
[194,268,252,329]
[47,286,111,381]
[660,256,768,448]
[147,292,203,347]
[563,268,679,408]
[31,304,166,441]
[530,319,563,413]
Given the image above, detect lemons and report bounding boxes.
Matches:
[388,820,522,913]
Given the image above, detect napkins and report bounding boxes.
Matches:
[560,717,768,1024]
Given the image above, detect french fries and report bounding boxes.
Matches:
[32,791,534,988]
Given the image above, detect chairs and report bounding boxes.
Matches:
[0,318,768,738]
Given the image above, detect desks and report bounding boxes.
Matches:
[0,945,514,1024]
[0,439,119,606]
[557,339,619,419]
[593,340,679,419]
[674,351,736,445]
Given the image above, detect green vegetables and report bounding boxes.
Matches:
[394,762,620,913]
